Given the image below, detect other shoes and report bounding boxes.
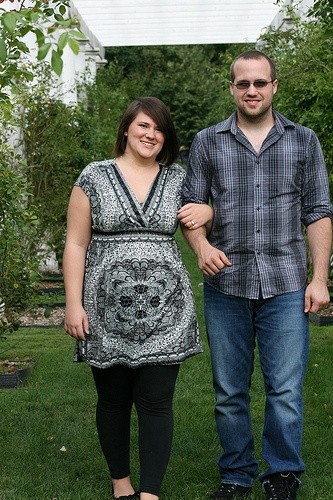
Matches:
[112,482,139,500]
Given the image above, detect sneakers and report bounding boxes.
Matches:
[209,482,252,500]
[264,471,299,500]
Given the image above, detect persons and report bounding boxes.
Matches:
[178,50,333,500]
[63,96,214,500]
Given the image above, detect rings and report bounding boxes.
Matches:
[191,220,195,225]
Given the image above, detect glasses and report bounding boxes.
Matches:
[232,79,275,88]
